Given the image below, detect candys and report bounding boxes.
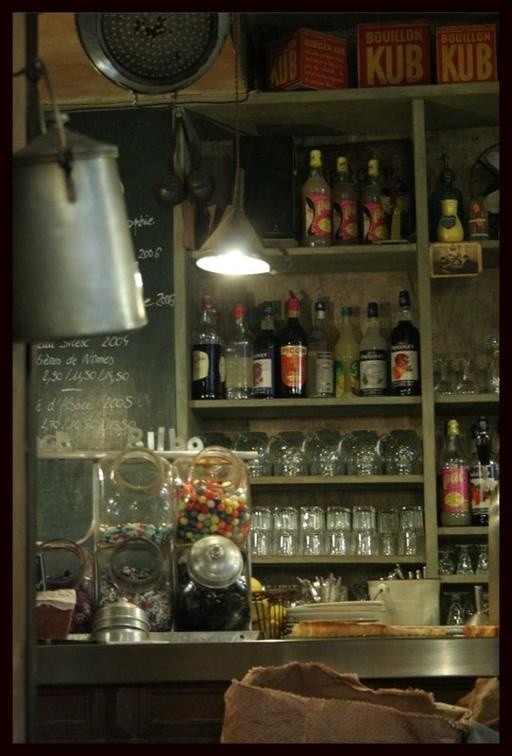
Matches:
[177,478,250,545]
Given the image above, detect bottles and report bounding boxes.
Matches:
[300,150,333,248]
[190,290,421,398]
[437,420,471,525]
[32,539,95,632]
[358,157,388,246]
[175,535,252,633]
[468,415,492,525]
[330,156,358,246]
[90,446,181,554]
[437,198,466,244]
[97,538,173,632]
[167,445,250,554]
[466,182,489,240]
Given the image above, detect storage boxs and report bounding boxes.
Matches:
[356,19,431,88]
[435,21,498,85]
[271,26,349,90]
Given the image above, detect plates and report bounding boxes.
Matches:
[285,600,388,626]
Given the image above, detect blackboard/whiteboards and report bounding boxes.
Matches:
[27,108,189,461]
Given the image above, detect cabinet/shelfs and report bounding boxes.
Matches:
[0,79,511,631]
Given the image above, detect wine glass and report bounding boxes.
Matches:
[438,543,488,577]
[442,590,489,626]
[432,332,501,396]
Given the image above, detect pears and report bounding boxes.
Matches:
[251,578,287,637]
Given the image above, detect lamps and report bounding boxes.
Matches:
[194,10,273,274]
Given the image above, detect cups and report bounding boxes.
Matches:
[201,429,423,476]
[249,505,424,557]
[299,580,371,603]
